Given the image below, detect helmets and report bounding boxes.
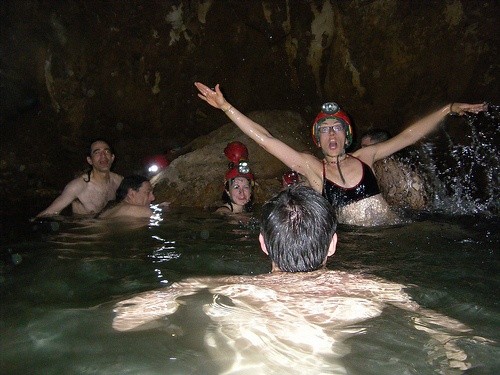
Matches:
[311,102,354,146]
[224,162,256,186]
[144,155,168,176]
[281,172,299,189]
[224,141,248,164]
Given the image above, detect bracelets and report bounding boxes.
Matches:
[450,101,457,115]
[224,105,233,114]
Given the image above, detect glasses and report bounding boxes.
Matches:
[317,124,345,133]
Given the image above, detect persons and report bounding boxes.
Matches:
[96,171,158,218]
[194,75,489,225]
[212,159,255,216]
[36,138,124,216]
[360,128,425,210]
[282,150,315,189]
[112,184,496,375]
